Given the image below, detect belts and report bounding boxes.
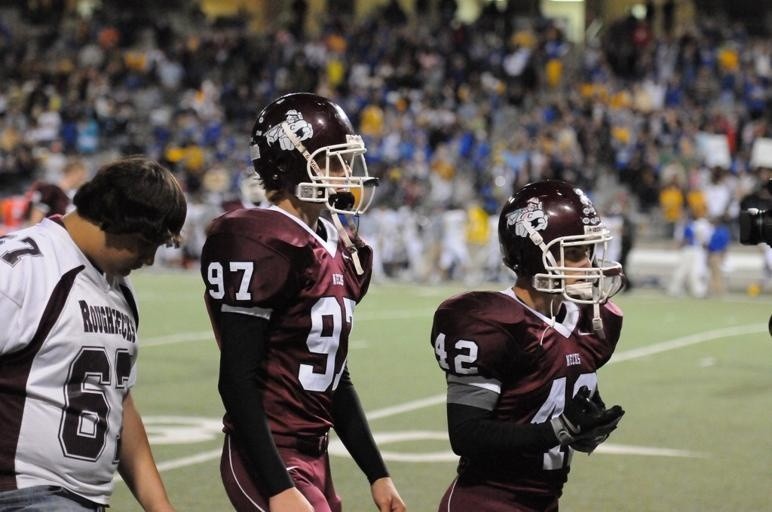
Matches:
[300,433,328,457]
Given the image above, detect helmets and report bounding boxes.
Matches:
[249,93,379,217]
[497,179,626,306]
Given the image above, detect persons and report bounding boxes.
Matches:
[1,0,772,300]
[200,93,408,512]
[431,178,627,511]
[0,156,187,512]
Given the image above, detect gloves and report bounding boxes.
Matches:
[551,386,625,456]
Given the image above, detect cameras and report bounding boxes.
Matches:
[739,207,772,249]
